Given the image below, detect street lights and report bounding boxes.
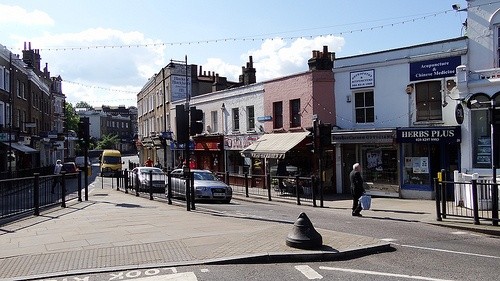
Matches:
[470,99,497,225]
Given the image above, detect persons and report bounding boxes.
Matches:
[176,155,185,168]
[87,162,93,183]
[350,163,366,216]
[145,157,161,168]
[52,160,68,194]
[275,158,286,194]
[366,152,382,168]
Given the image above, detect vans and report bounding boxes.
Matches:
[75,157,91,170]
[99,149,123,177]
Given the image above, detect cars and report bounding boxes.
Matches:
[164,169,232,203]
[59,162,82,178]
[129,167,167,191]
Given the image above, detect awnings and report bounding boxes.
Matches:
[239,132,311,160]
[3,142,39,154]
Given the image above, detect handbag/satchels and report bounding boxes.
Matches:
[358,192,371,210]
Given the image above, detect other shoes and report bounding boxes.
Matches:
[352,212,362,217]
